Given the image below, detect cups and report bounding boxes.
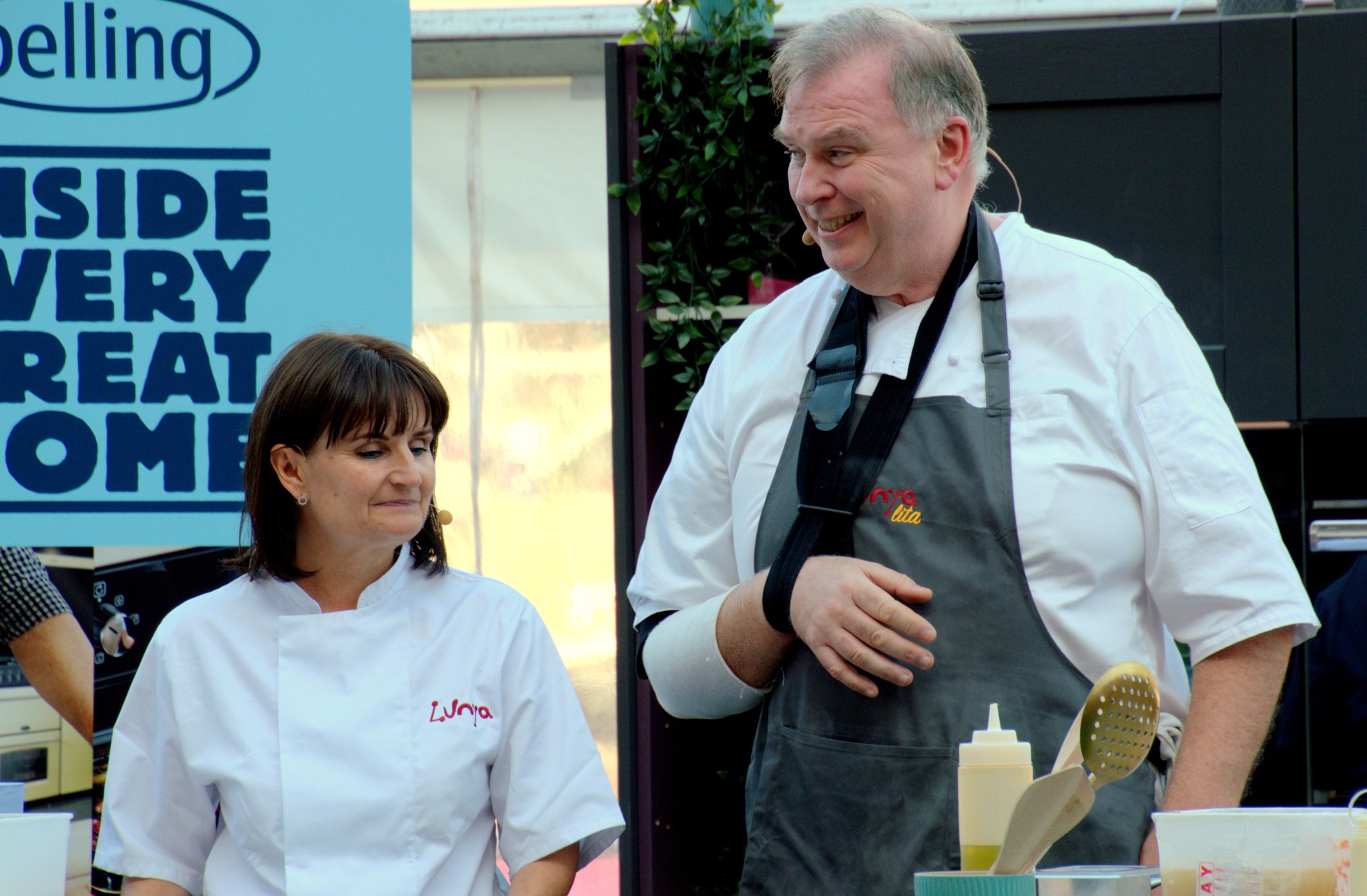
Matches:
[1149,807,1367,895]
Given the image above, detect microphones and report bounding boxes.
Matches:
[802,229,816,245]
[429,504,453,525]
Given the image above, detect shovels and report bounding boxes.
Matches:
[990,764,1087,874]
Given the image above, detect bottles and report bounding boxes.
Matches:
[957,702,1033,872]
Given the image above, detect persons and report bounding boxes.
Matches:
[0,547,96,749]
[626,4,1325,896]
[92,330,627,896]
